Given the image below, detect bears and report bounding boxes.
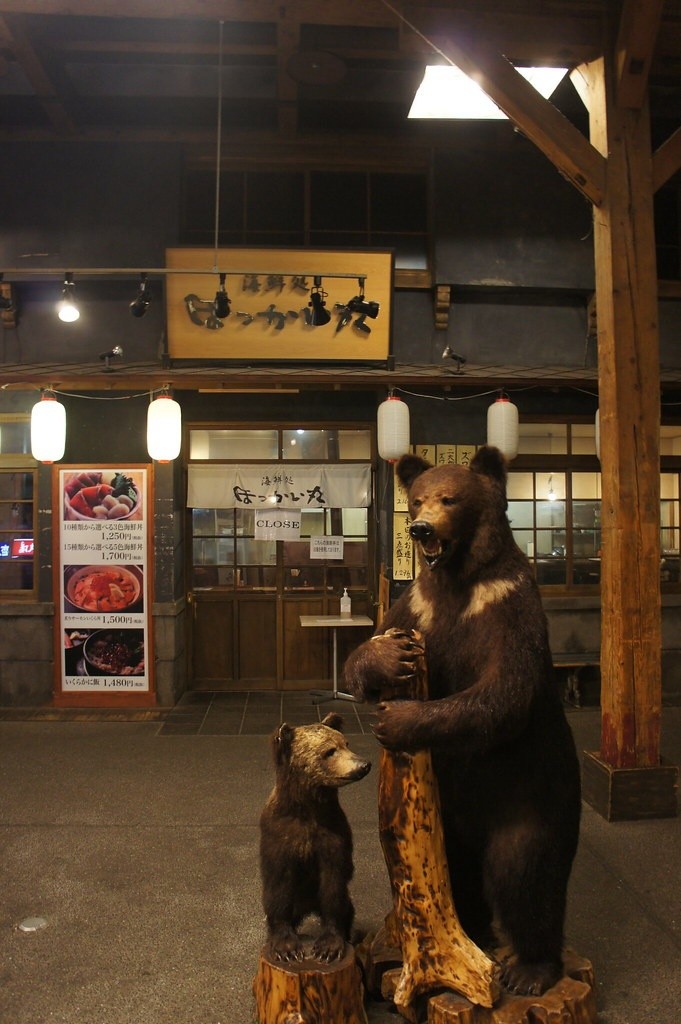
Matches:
[344,444,584,996]
[257,712,373,962]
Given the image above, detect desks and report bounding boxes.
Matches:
[299,615,374,706]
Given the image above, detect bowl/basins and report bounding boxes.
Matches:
[65,476,141,521]
[83,628,144,675]
[64,631,89,655]
[63,564,143,614]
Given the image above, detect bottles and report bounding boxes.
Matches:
[340,588,351,619]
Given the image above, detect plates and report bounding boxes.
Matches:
[84,660,110,677]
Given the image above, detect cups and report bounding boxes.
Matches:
[233,570,240,585]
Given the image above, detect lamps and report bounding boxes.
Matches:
[55,271,379,322]
